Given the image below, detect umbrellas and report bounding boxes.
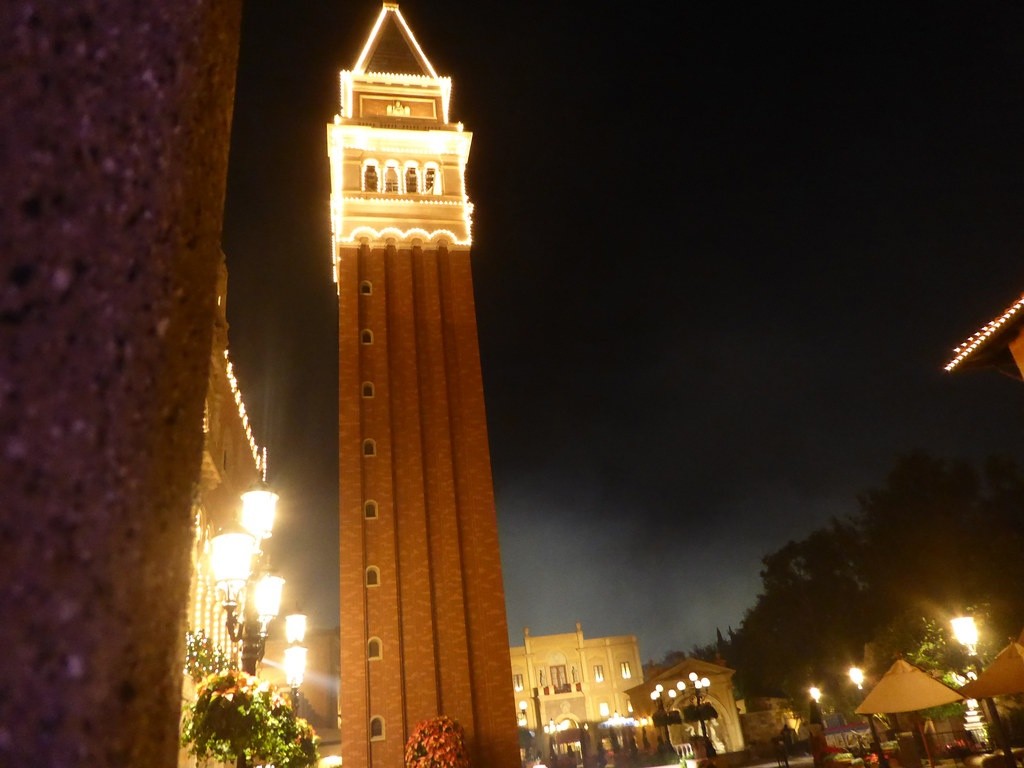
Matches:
[854,658,964,762]
[958,641,1024,698]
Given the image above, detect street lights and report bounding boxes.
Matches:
[205,481,288,768]
[282,610,312,716]
[847,666,891,768]
[949,615,1017,768]
[689,671,717,757]
[650,684,677,741]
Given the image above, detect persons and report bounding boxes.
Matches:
[780,724,796,758]
[797,721,811,756]
